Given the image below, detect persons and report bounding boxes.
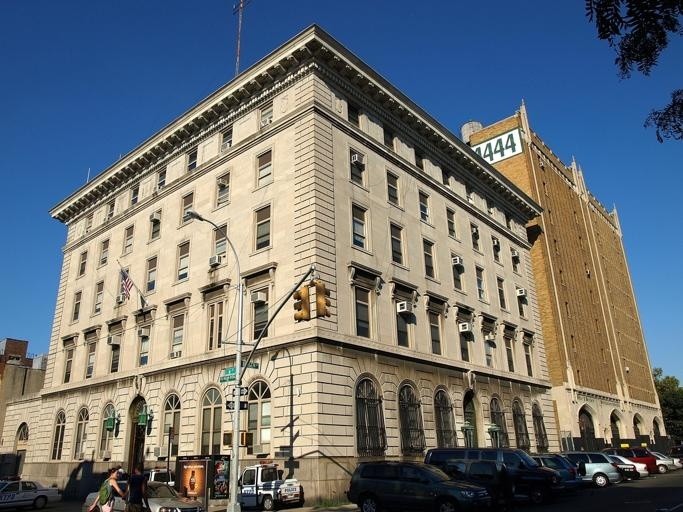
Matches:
[116,465,125,473]
[84,467,124,512]
[118,463,151,511]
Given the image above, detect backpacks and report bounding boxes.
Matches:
[100,482,109,506]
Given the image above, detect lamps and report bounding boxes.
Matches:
[106,408,120,431]
[138,404,153,426]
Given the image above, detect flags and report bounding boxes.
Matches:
[120,270,133,300]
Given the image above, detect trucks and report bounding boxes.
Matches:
[234,463,303,509]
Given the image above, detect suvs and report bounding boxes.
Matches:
[346,460,492,510]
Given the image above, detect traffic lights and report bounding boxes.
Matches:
[291,287,310,322]
[313,280,331,317]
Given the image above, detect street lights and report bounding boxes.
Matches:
[186,210,243,512]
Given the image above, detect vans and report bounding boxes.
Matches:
[601,448,657,474]
[423,447,560,501]
[560,451,622,487]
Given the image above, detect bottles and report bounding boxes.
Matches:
[189,470,196,491]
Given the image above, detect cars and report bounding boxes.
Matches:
[610,454,649,477]
[607,456,639,481]
[649,452,673,474]
[81,479,203,512]
[529,454,577,493]
[653,452,683,471]
[0,480,62,511]
